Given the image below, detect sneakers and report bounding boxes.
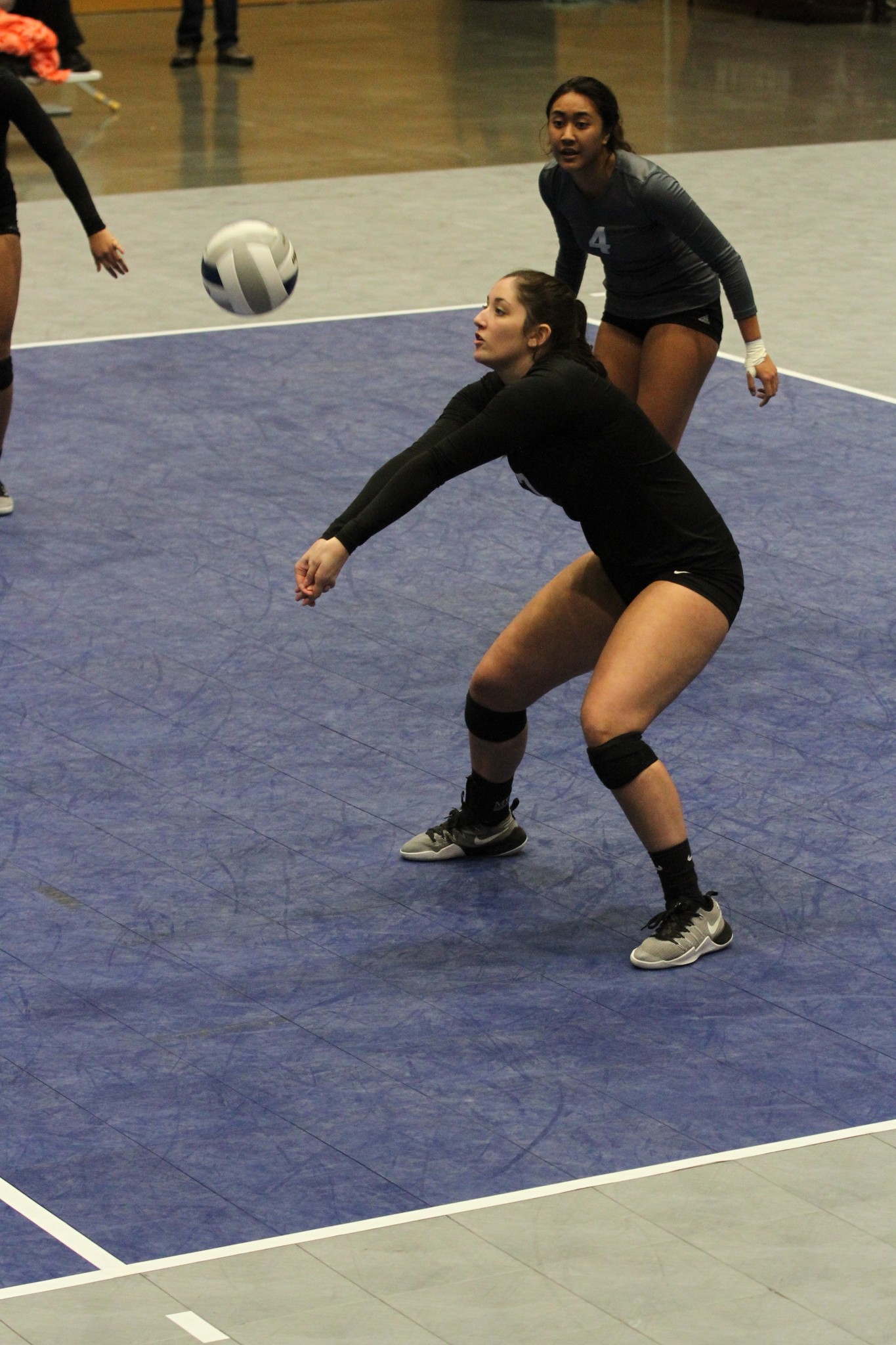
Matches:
[397,792,526,860]
[630,887,732,968]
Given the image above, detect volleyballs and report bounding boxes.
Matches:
[201,219,298,317]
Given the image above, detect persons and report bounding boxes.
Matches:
[538,76,779,451]
[169,0,254,68]
[0,65,129,516]
[293,268,743,969]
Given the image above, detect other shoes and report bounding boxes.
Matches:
[214,40,254,67]
[169,47,200,68]
[0,479,15,515]
